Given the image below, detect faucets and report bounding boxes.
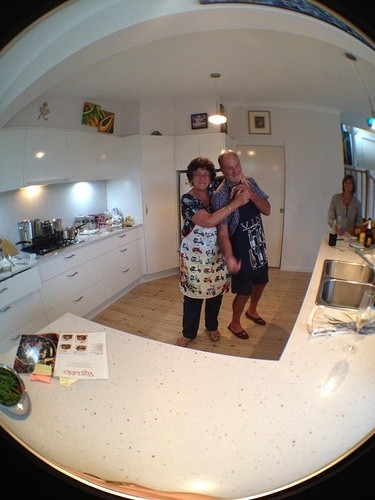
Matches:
[353,249,375,272]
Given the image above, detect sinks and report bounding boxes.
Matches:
[322,259,375,285]
[315,278,375,311]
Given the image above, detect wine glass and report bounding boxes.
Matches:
[343,290,375,354]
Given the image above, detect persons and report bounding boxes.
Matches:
[328,174,363,232]
[177,157,250,347]
[212,148,271,339]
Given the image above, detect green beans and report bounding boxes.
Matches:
[0,366,23,406]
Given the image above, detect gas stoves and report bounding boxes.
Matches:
[21,239,84,255]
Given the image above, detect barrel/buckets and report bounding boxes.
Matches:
[75,216,86,230]
[86,216,96,229]
[17,218,61,246]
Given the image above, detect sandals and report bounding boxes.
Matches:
[176,336,193,347]
[208,327,220,342]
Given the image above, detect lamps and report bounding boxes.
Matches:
[207,73,227,124]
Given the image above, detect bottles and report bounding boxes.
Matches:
[360,218,366,244]
[364,218,372,248]
[328,219,337,247]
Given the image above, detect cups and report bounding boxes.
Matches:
[0,363,29,415]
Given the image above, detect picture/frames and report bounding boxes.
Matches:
[248,110,272,135]
[191,113,208,130]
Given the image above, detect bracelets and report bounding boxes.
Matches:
[227,205,233,212]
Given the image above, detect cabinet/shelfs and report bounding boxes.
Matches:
[0,129,121,193]
[175,133,233,170]
[0,228,145,353]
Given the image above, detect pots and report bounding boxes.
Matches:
[15,234,57,246]
[55,223,86,240]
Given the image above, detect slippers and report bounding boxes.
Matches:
[227,323,249,340]
[245,312,266,325]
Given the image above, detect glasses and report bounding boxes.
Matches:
[192,173,211,179]
